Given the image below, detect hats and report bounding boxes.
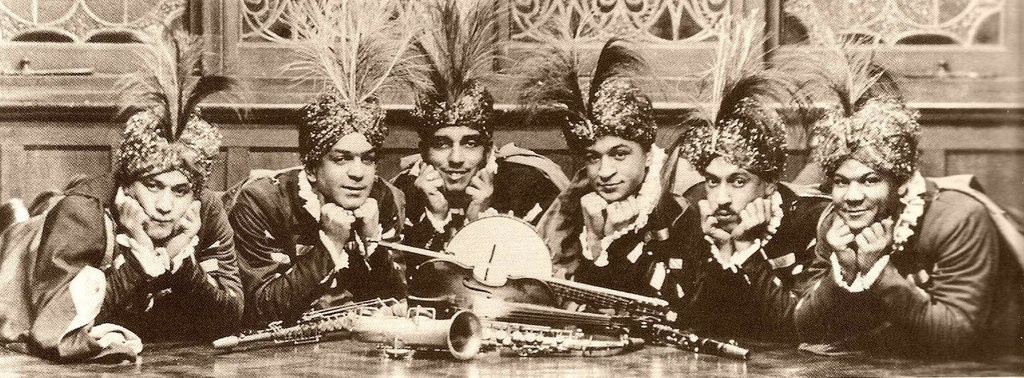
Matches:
[385,1,521,147]
[781,32,918,187]
[509,10,674,155]
[662,18,814,191]
[274,0,434,165]
[113,25,251,198]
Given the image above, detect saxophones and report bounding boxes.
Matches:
[348,311,645,361]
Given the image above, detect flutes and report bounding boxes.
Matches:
[212,297,408,353]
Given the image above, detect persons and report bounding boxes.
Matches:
[536,77,705,311]
[218,87,409,331]
[790,94,1024,355]
[388,76,570,286]
[0,101,245,365]
[668,114,835,348]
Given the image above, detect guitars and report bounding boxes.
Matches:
[449,214,686,326]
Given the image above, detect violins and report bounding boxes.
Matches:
[411,258,656,333]
